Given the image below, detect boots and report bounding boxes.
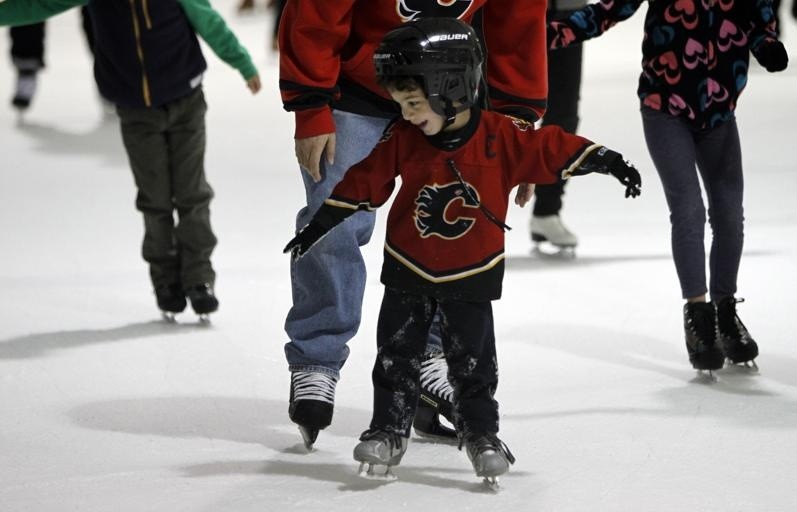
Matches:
[10,72,40,109]
[288,367,336,426]
[682,295,758,367]
[530,213,576,246]
[151,283,218,312]
[354,398,416,464]
[454,403,510,474]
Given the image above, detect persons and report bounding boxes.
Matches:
[8,18,45,110]
[0,1,262,312]
[282,16,644,478]
[524,0,593,250]
[275,0,546,430]
[546,1,791,372]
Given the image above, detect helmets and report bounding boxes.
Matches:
[375,18,483,115]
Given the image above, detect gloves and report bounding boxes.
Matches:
[592,144,641,198]
[282,213,346,260]
